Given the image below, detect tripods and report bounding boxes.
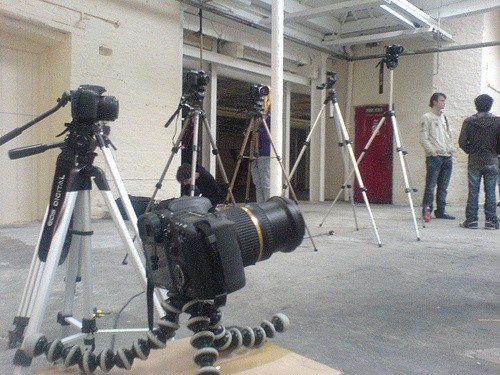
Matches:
[123,58,426,267]
[9,123,169,375]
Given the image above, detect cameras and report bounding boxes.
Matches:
[384,45,404,58]
[186,69,210,91]
[327,71,336,87]
[69,85,119,123]
[139,194,306,301]
[250,84,269,101]
